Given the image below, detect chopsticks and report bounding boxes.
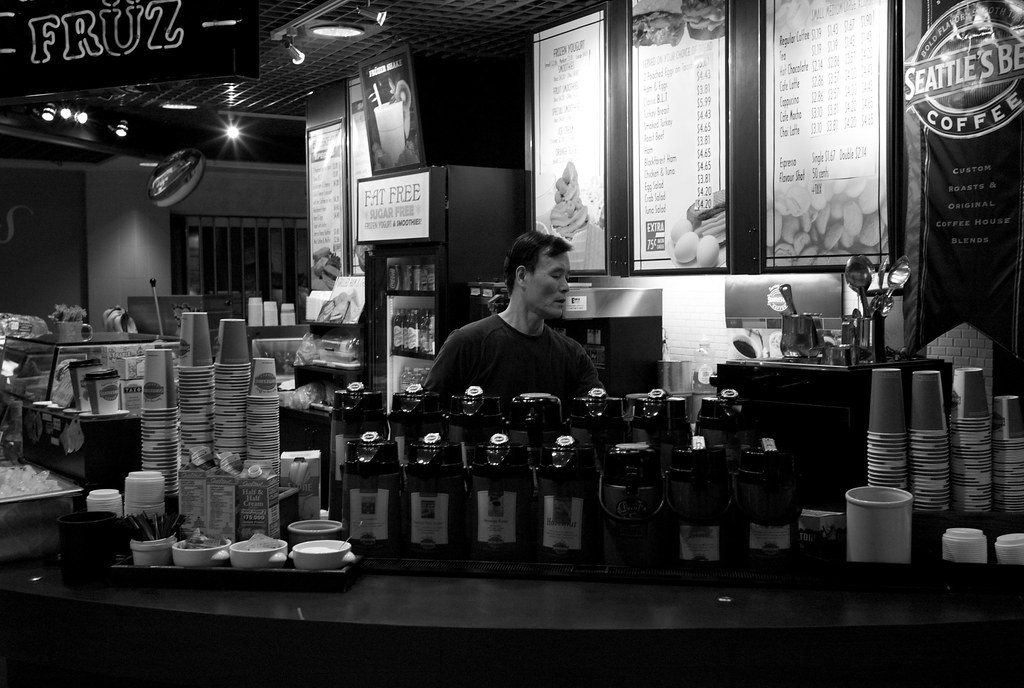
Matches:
[123,511,186,541]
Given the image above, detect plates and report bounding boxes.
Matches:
[33,402,51,407]
[63,409,82,413]
[86,472,166,520]
[994,533,1024,566]
[942,528,987,564]
[78,410,129,417]
[47,404,65,409]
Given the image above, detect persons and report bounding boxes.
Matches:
[425,230,607,396]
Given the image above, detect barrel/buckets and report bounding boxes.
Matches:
[843,314,887,364]
[781,315,818,357]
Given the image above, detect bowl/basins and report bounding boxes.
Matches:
[0,460,83,561]
[172,539,231,567]
[288,520,343,548]
[248,297,295,325]
[290,540,352,571]
[229,539,288,569]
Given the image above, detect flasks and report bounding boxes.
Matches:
[328,390,795,571]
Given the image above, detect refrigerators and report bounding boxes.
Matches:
[357,165,528,438]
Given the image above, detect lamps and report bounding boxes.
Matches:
[42,103,88,124]
[357,0,387,26]
[116,120,128,137]
[285,26,305,64]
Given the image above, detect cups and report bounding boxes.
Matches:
[846,486,914,564]
[54,321,93,342]
[374,101,405,169]
[130,535,177,566]
[57,511,118,590]
[85,369,119,413]
[140,312,280,504]
[68,359,103,411]
[867,369,1024,513]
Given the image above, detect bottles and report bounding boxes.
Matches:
[391,308,435,354]
[401,366,432,395]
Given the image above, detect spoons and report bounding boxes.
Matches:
[852,296,893,317]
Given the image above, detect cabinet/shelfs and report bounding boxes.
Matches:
[281,321,367,510]
